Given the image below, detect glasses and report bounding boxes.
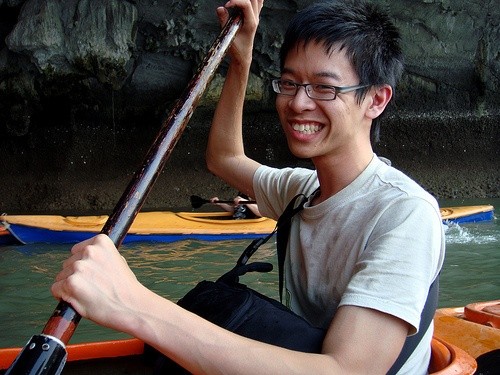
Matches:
[270,77,371,101]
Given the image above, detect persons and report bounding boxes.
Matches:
[51,0,447,375]
[209,189,264,219]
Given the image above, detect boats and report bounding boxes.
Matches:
[0,203,495,247]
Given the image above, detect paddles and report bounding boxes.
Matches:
[3,9,243,375]
[189,195,257,209]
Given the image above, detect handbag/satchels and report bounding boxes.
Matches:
[144,270,326,373]
